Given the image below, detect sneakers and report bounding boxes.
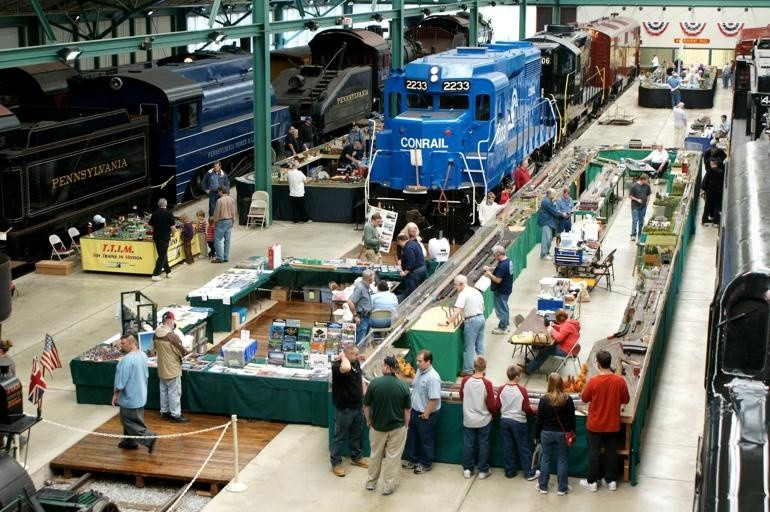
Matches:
[492,328,508,334]
[402,460,420,469]
[414,465,432,474]
[166,272,172,279]
[151,275,163,281]
[527,469,617,496]
[463,468,471,479]
[478,467,492,480]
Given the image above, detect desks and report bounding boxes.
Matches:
[638,65,718,108]
[232,129,374,225]
[684,124,713,156]
[72,255,443,427]
[80,217,207,276]
[356,127,702,484]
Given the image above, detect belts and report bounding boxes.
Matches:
[465,314,482,320]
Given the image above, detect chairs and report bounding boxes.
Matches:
[246,192,269,232]
[49,234,75,262]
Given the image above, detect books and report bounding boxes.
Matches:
[174,323,332,380]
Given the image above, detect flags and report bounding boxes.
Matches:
[40,333,62,377]
[28,356,47,405]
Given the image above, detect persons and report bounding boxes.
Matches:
[483,245,514,334]
[154,311,192,422]
[517,311,581,376]
[402,349,441,475]
[556,187,574,235]
[284,117,367,224]
[370,281,398,338]
[515,158,531,190]
[397,234,427,297]
[439,274,486,377]
[363,355,412,493]
[460,356,496,480]
[641,143,670,186]
[0,339,28,451]
[629,173,651,241]
[535,372,575,493]
[538,188,557,261]
[673,102,687,149]
[578,351,628,491]
[149,160,236,281]
[363,215,385,265]
[701,114,731,228]
[331,344,369,477]
[639,54,735,108]
[347,270,374,344]
[396,222,419,261]
[499,185,512,204]
[478,191,504,227]
[112,333,156,455]
[497,364,541,481]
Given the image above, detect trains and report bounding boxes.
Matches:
[365,12,642,243]
[0,11,495,262]
[691,25,766,512]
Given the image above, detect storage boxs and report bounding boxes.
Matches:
[35,259,76,275]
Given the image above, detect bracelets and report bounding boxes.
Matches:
[447,322,449,324]
[354,314,358,317]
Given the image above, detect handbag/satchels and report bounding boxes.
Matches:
[564,431,577,448]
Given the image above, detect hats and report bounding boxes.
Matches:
[162,311,175,319]
[384,356,400,374]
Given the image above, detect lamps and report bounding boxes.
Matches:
[58,0,496,62]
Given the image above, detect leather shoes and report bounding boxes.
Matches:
[117,441,140,449]
[350,458,369,468]
[458,371,472,377]
[148,435,156,454]
[168,415,189,423]
[210,258,224,262]
[162,413,169,419]
[332,462,345,476]
